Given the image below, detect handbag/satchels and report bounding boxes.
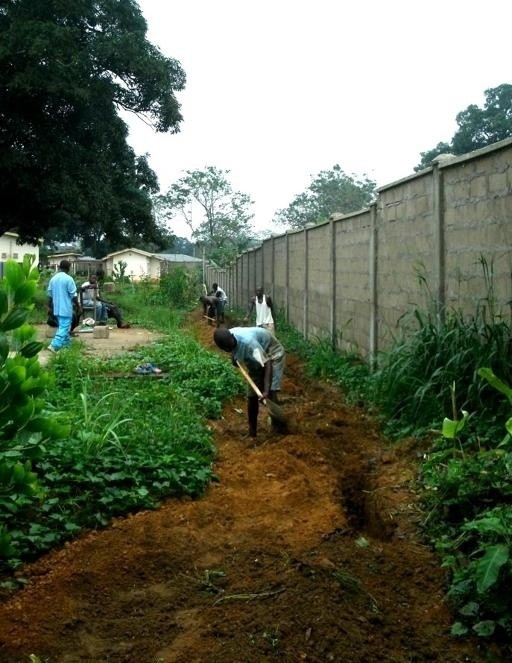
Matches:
[47,313,59,328]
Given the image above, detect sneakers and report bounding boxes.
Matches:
[47,344,57,355]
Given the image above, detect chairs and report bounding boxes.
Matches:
[77,286,101,328]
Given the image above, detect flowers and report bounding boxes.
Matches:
[82,317,95,326]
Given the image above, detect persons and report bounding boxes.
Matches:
[94,269,132,328]
[199,294,222,324]
[214,326,284,436]
[208,282,227,319]
[244,285,277,337]
[81,273,108,324]
[47,258,84,354]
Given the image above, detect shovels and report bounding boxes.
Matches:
[204,315,221,325]
[236,361,289,433]
